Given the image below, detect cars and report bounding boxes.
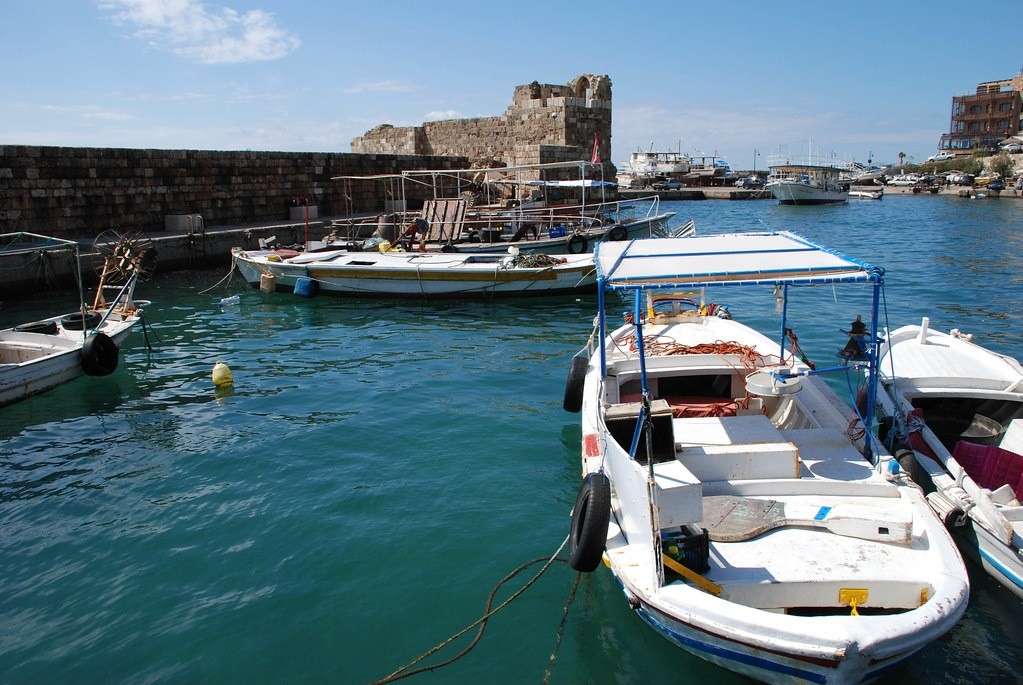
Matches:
[888,177,916,186]
[735,177,755,190]
[1002,142,1021,152]
[749,178,767,190]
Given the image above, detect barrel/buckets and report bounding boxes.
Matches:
[294,277,313,296]
[378,217,399,243]
[260,274,274,294]
[212,361,233,388]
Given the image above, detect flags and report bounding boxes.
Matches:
[591,135,600,169]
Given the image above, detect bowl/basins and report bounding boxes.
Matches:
[959,414,1003,446]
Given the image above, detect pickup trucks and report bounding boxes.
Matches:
[929,152,956,161]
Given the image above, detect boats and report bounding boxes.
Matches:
[324,158,679,258]
[230,175,608,297]
[764,136,854,205]
[840,149,884,198]
[0,229,159,408]
[621,143,725,175]
[859,315,1023,599]
[563,230,970,685]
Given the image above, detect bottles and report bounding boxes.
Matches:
[219,296,240,307]
[888,458,899,475]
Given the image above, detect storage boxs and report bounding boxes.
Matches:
[953,440,1023,501]
[548,225,566,239]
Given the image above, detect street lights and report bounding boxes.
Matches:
[754,148,760,176]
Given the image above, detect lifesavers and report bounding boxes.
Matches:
[440,245,459,254]
[563,355,588,413]
[12,321,59,335]
[80,333,120,378]
[608,225,628,241]
[568,473,614,574]
[468,230,481,244]
[566,233,589,254]
[61,310,102,332]
